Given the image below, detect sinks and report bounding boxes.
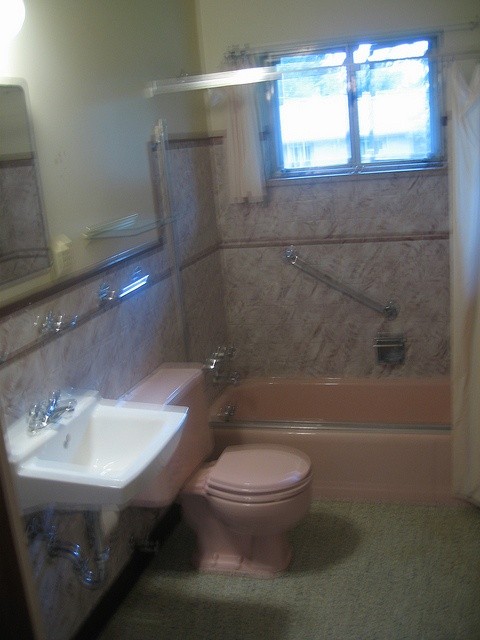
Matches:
[17,391,192,506]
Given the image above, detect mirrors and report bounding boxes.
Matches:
[0,85,54,294]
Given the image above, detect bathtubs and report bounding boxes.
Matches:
[208,376,451,507]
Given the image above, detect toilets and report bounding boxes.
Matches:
[116,361,312,579]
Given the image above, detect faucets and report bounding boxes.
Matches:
[206,344,240,388]
[26,388,78,436]
